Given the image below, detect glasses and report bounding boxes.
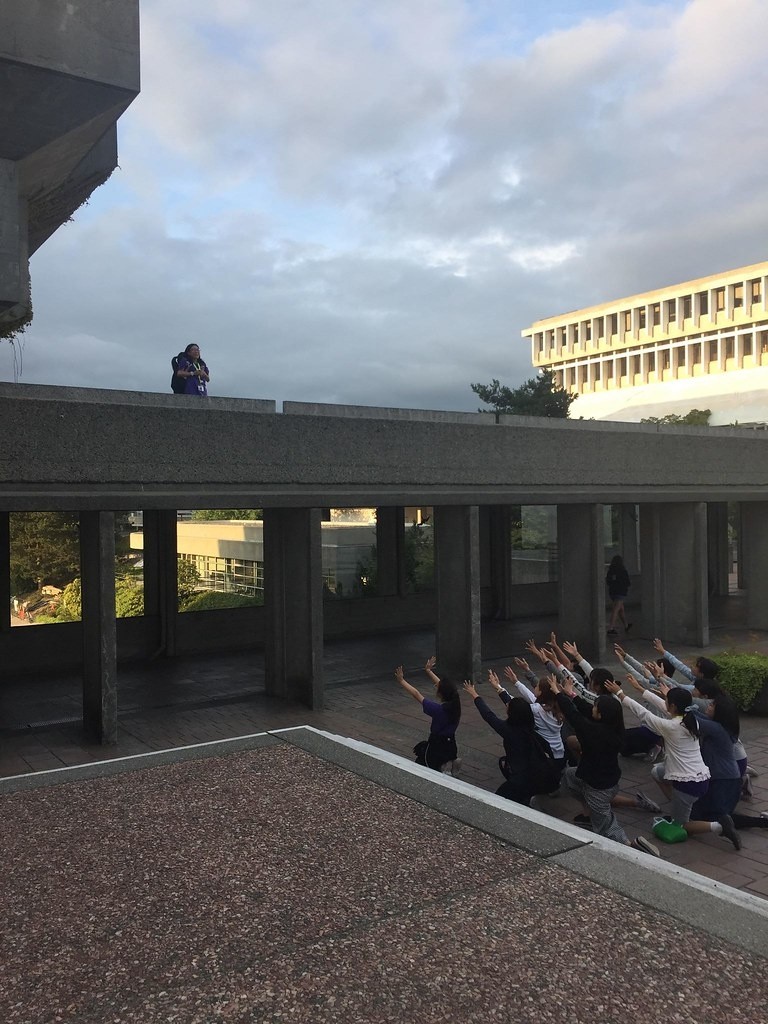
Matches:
[433,684,438,692]
[188,348,200,352]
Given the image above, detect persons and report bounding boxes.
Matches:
[605,556,633,636]
[395,657,463,781]
[462,631,760,851]
[176,344,212,395]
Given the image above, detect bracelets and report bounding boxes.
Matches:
[495,686,502,693]
[616,689,623,696]
[190,371,194,376]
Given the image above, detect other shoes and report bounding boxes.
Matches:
[643,745,662,763]
[574,813,593,831]
[548,784,561,797]
[742,774,753,801]
[631,836,660,858]
[760,812,768,820]
[658,737,665,758]
[453,758,462,777]
[625,623,632,633]
[745,765,759,777]
[717,814,741,850]
[636,791,661,813]
[529,796,536,807]
[441,761,452,775]
[662,799,672,814]
[608,629,619,635]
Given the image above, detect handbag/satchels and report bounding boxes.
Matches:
[652,816,688,843]
